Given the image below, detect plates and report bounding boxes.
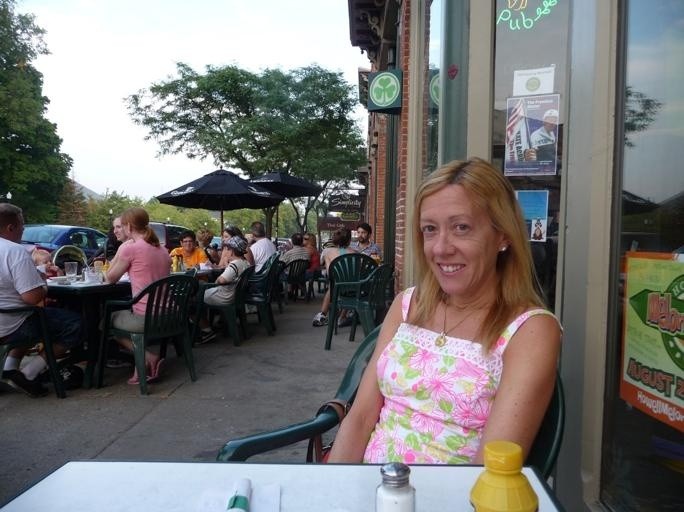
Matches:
[49,276,82,282]
[171,272,186,275]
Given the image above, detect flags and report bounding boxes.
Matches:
[505,98,525,149]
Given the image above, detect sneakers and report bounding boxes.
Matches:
[8,372,50,398]
[194,329,216,346]
[1,370,20,392]
[147,354,165,383]
[127,366,139,385]
[312,312,329,326]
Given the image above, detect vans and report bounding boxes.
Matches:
[148,221,190,254]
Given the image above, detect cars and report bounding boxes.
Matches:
[209,237,222,249]
[19,224,108,259]
[276,238,292,248]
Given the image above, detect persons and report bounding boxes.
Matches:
[280,233,311,301]
[313,228,361,327]
[531,108,559,161]
[325,157,562,464]
[0,199,86,399]
[101,207,173,386]
[524,147,537,162]
[31,249,63,280]
[105,214,137,281]
[187,237,252,346]
[218,225,256,266]
[249,219,278,274]
[303,232,320,304]
[169,230,213,285]
[349,222,382,264]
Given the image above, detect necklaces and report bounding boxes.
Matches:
[434,294,479,347]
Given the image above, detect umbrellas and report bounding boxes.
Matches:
[245,168,324,251]
[154,165,286,250]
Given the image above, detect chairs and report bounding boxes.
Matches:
[267,261,286,331]
[317,278,329,294]
[244,252,281,336]
[280,259,310,306]
[324,262,394,352]
[301,277,319,303]
[189,264,256,348]
[216,321,567,481]
[94,273,200,395]
[253,252,281,276]
[330,253,380,336]
[0,303,67,400]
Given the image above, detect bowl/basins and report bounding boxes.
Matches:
[85,272,103,283]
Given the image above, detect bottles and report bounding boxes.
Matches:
[373,459,417,512]
[468,440,540,512]
[171,253,180,273]
[370,252,377,259]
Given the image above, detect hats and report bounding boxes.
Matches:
[247,222,266,236]
[225,236,249,254]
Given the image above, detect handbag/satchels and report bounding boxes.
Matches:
[307,399,351,464]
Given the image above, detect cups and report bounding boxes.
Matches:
[65,262,77,282]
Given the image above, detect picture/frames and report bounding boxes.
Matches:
[504,92,561,177]
